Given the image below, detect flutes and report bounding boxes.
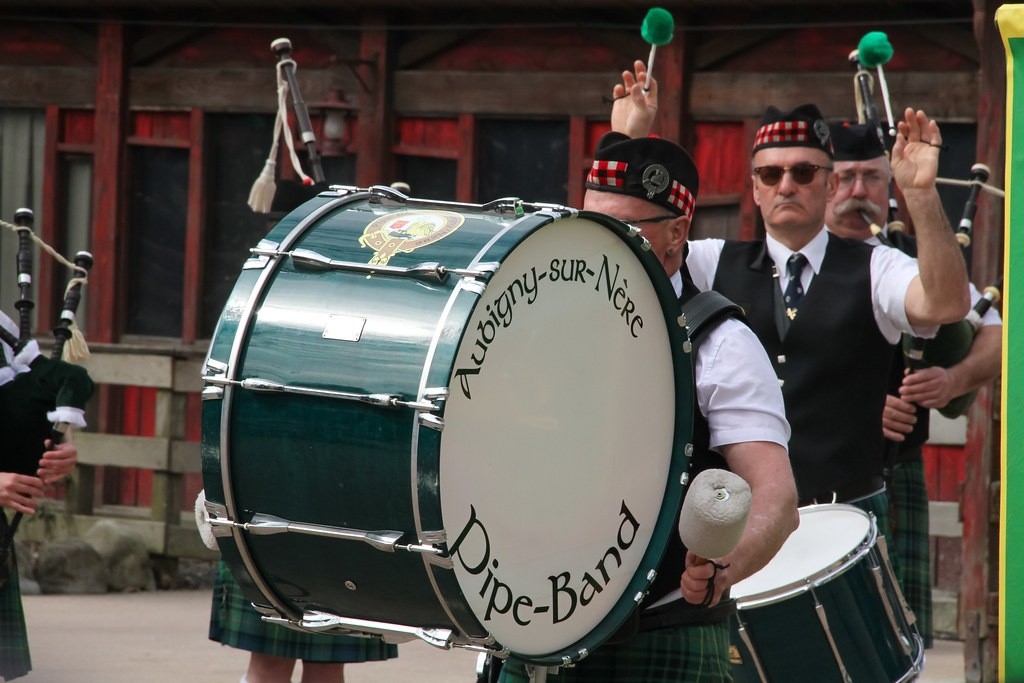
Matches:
[0,205,97,553]
[855,160,1002,484]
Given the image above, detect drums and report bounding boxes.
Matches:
[729,503,925,683]
[199,182,696,662]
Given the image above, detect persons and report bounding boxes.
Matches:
[826,117,1003,649]
[610,61,970,598]
[209,554,397,683]
[488,129,799,683]
[0,310,77,683]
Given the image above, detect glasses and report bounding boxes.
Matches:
[834,168,892,189]
[750,161,833,186]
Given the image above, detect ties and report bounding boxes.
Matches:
[783,252,808,322]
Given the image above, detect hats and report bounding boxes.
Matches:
[829,121,886,163]
[585,131,699,223]
[752,104,835,160]
